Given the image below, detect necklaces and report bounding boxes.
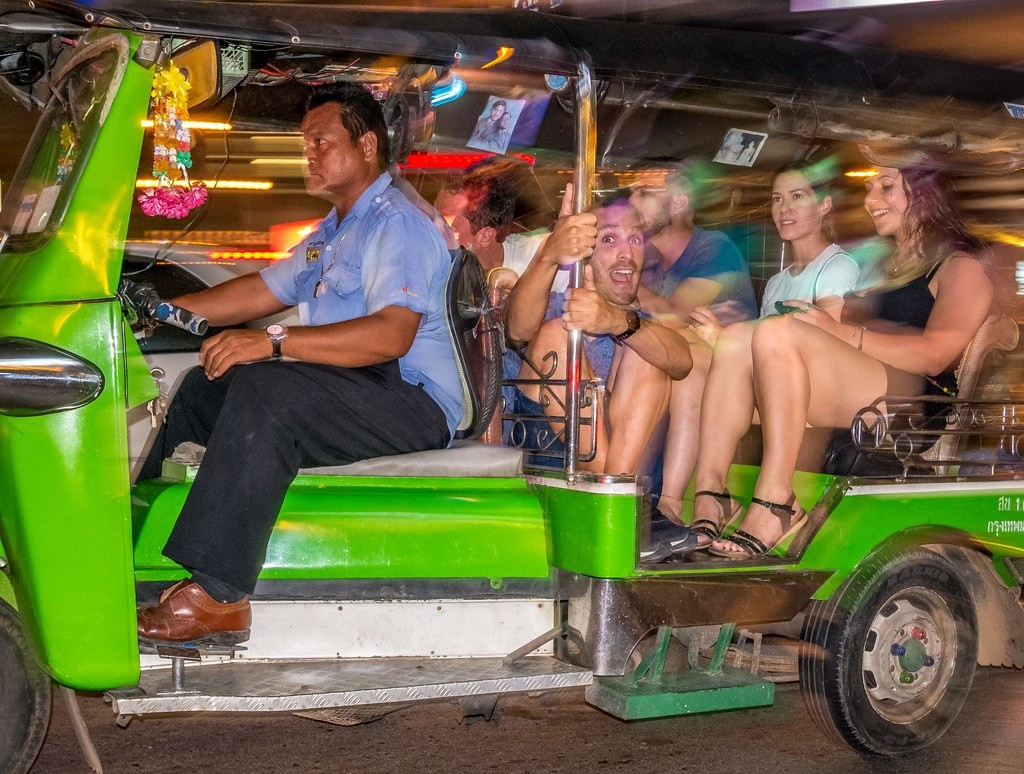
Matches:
[891,249,925,271]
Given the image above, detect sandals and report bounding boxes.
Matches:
[708,490,808,558]
[689,487,744,550]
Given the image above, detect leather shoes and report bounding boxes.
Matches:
[137,578,253,646]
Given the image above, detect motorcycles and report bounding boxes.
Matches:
[0,0,1023,774]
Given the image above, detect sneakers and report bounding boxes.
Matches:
[640,490,697,562]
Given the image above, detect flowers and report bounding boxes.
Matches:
[136,60,210,219]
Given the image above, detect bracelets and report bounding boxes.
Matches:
[849,325,867,352]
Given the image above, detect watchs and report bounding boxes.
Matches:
[612,308,640,347]
[266,323,287,359]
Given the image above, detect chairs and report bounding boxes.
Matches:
[301,249,530,477]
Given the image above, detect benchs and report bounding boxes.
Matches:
[821,321,1024,477]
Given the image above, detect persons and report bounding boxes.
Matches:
[472,100,511,155]
[132,80,465,649]
[725,137,755,165]
[690,149,995,557]
[502,182,693,494]
[434,169,570,293]
[469,154,556,242]
[652,158,861,528]
[628,160,757,326]
